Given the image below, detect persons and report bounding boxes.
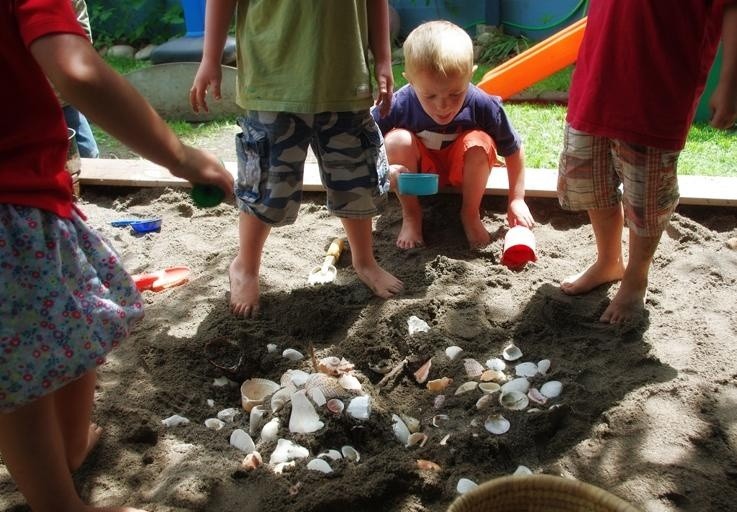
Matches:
[1,1,236,512]
[44,0,100,158]
[371,19,537,251]
[555,0,737,327]
[189,0,406,321]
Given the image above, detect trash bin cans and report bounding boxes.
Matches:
[181,1,205,37]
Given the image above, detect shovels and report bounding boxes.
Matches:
[130,267,190,291]
[308,239,343,283]
